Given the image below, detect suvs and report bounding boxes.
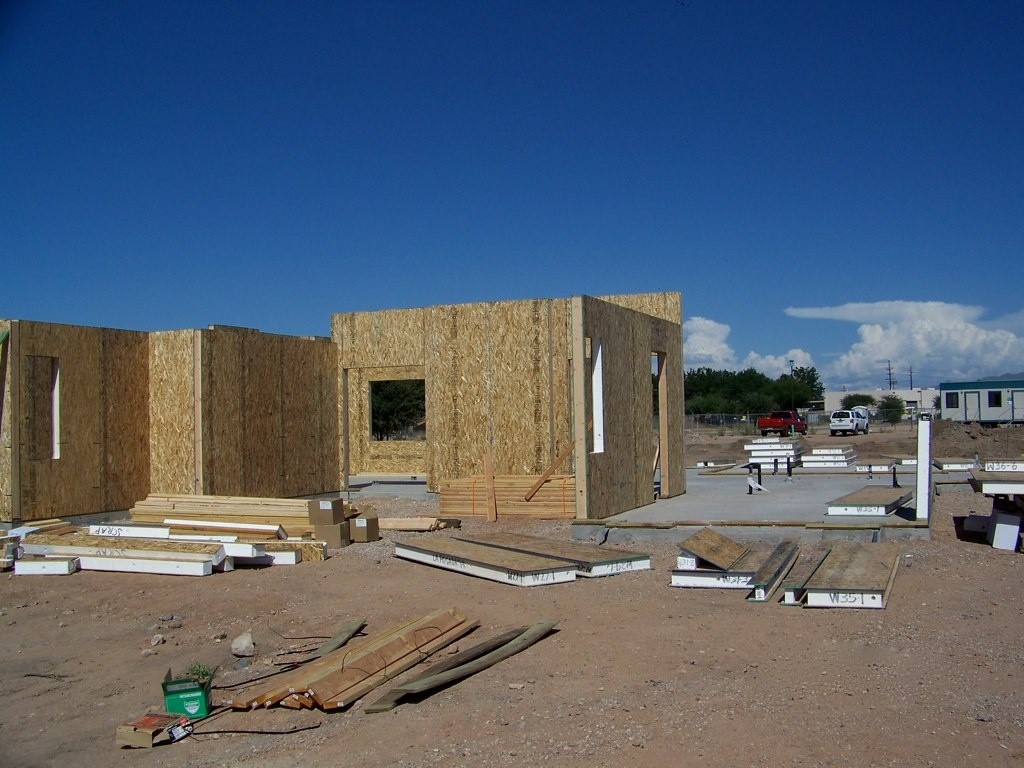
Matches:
[830,410,869,436]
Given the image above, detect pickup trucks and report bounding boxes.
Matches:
[758,410,808,436]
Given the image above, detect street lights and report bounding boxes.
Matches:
[788,358,794,411]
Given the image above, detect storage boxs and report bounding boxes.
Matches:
[160,667,223,719]
